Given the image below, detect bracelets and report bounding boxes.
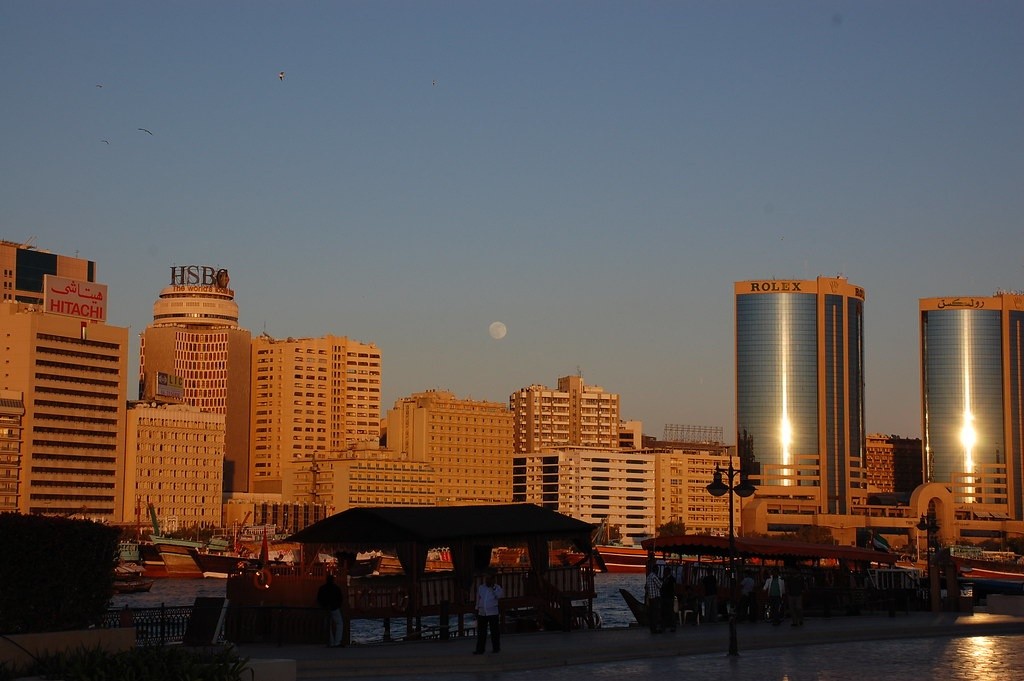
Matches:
[493,585,495,590]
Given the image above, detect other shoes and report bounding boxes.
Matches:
[334,644,345,648]
[493,649,500,653]
[473,651,483,654]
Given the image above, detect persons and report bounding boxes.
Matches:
[644,567,807,636]
[469,573,505,654]
[314,574,346,648]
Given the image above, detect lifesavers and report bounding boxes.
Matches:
[825,569,834,585]
[254,568,273,589]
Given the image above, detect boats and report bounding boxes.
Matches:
[562,543,742,575]
[381,552,591,579]
[148,511,255,578]
[113,577,154,594]
[185,519,384,581]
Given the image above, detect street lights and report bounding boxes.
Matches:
[706,454,759,654]
